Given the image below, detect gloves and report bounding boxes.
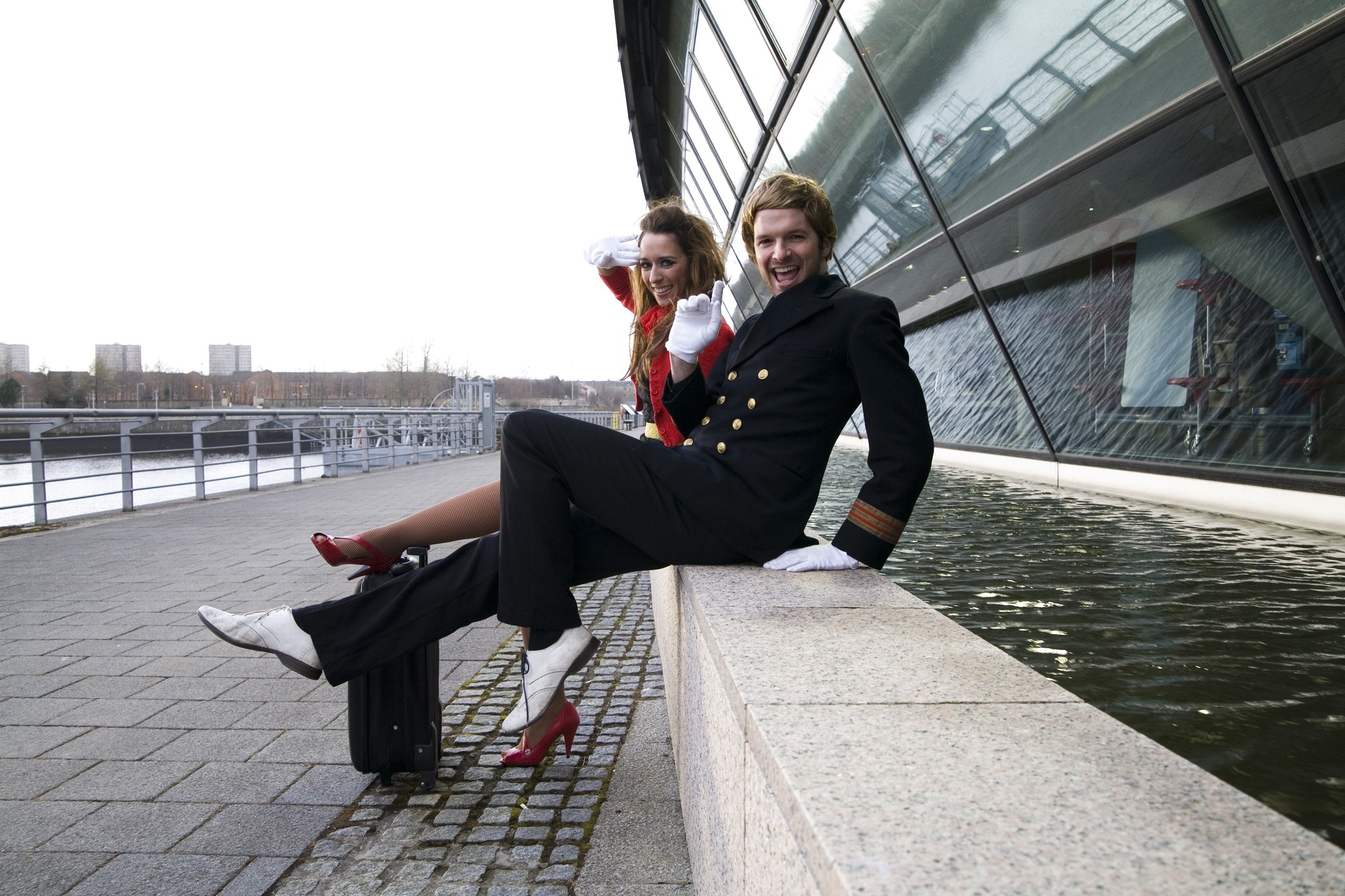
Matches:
[763,544,859,572]
[665,280,725,364]
[585,234,641,269]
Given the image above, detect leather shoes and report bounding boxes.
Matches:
[501,625,601,734]
[196,605,323,680]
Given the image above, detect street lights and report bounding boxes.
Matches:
[91,390,95,409]
[20,390,24,409]
[300,383,303,407]
[251,381,258,408]
[136,383,144,408]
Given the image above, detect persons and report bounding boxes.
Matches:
[312,196,737,767]
[194,176,935,735]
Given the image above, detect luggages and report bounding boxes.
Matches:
[347,544,444,792]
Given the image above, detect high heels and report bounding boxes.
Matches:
[310,532,403,581]
[499,701,580,766]
